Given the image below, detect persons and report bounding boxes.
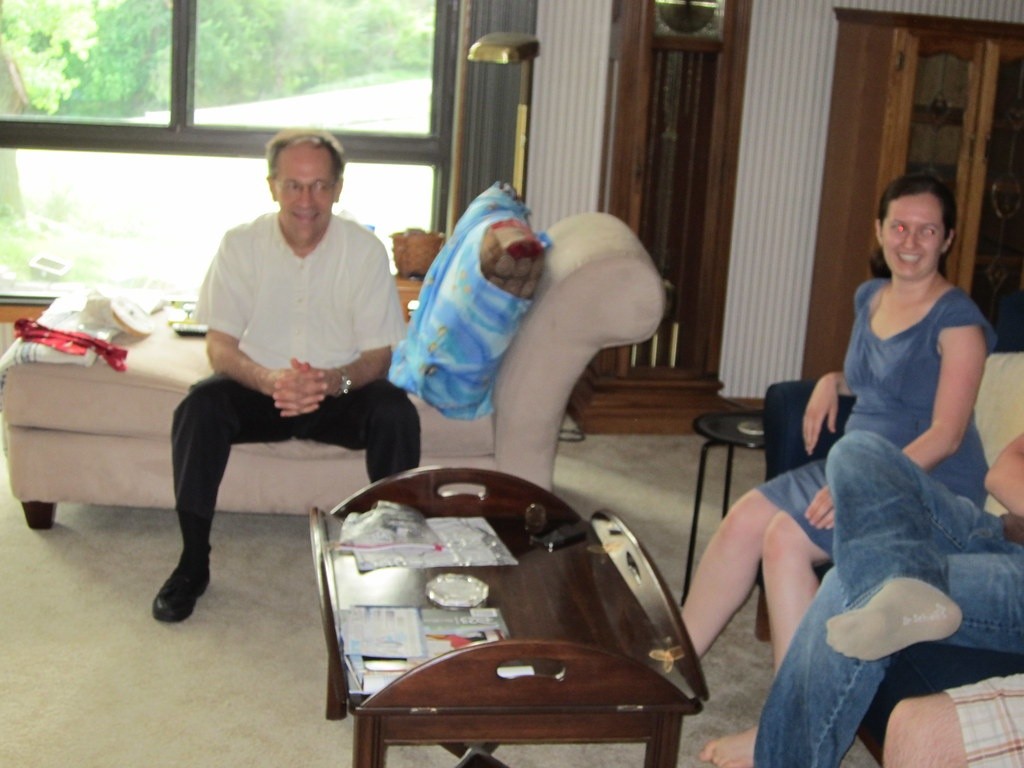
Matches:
[678,173,1000,767]
[752,430,1024,768]
[883,673,1023,768]
[152,130,423,622]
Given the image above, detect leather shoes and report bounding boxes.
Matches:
[152,551,210,621]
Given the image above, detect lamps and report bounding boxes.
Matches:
[467,30,542,203]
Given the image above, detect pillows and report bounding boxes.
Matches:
[386,178,553,423]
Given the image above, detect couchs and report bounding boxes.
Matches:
[758,352,1024,768]
[0,211,671,529]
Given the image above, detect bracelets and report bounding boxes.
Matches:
[332,368,351,398]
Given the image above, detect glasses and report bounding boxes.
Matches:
[886,219,942,239]
[275,177,339,196]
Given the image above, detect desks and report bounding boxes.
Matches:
[306,464,709,768]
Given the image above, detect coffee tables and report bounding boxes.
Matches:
[681,411,765,608]
